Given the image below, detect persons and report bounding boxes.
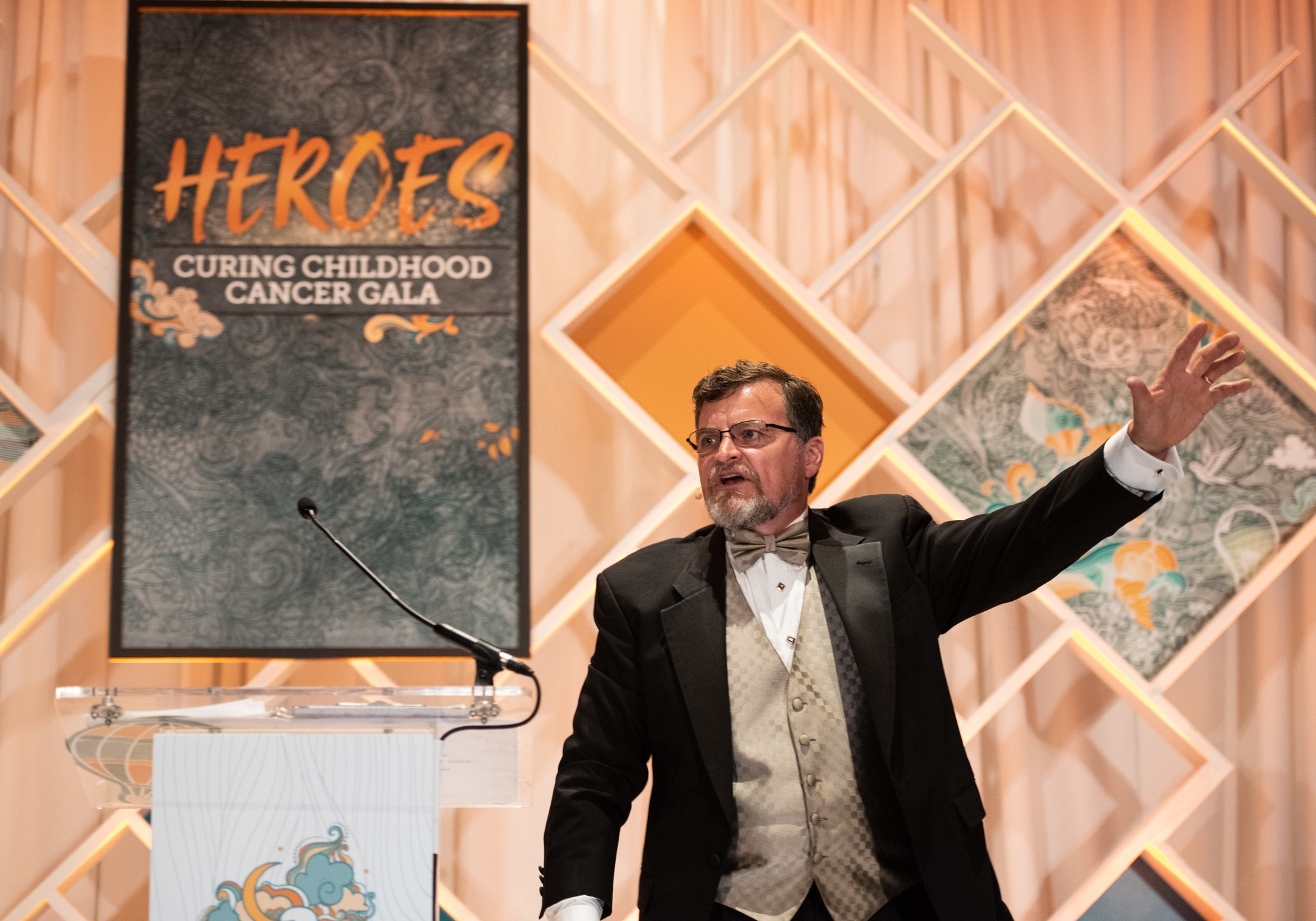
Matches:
[539,320,1254,921]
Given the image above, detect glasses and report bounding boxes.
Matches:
[686,419,797,454]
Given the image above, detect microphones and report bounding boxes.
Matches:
[296,495,534,676]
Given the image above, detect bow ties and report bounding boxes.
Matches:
[724,515,811,572]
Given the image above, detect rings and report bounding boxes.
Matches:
[1202,373,1213,386]
